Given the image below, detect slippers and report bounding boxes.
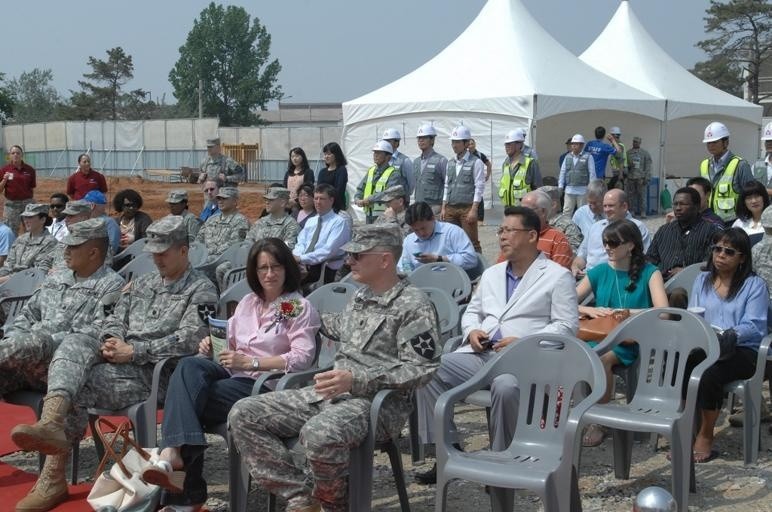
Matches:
[667,448,720,464]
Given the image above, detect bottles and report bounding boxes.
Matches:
[402,252,413,272]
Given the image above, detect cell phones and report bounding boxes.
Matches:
[480,340,491,346]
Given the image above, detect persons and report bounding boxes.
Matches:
[200,123,490,294]
[414,205,579,493]
[1,145,199,332]
[11,215,219,512]
[143,238,321,511]
[227,223,442,512]
[499,121,772,462]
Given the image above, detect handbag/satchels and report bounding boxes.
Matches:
[86,419,161,512]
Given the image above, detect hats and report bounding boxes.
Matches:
[142,215,187,253]
[20,203,49,216]
[633,136,643,142]
[165,188,189,205]
[216,187,238,199]
[339,223,404,252]
[261,186,291,200]
[58,218,108,246]
[61,191,106,215]
[205,139,221,147]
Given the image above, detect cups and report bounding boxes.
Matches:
[687,306,705,319]
[8,173,13,180]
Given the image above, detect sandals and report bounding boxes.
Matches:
[582,422,609,448]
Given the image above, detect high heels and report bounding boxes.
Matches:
[142,461,187,492]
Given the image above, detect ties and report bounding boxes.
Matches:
[305,216,323,254]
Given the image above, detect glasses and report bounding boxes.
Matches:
[496,227,533,235]
[343,251,385,260]
[712,244,742,256]
[49,204,64,208]
[204,188,215,193]
[603,239,632,248]
[257,264,287,271]
[122,203,139,207]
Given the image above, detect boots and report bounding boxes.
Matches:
[16,451,68,511]
[10,394,70,451]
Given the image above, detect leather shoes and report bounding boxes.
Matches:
[415,445,464,484]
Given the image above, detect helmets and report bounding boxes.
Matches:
[565,134,585,146]
[415,124,436,138]
[609,126,621,135]
[448,125,472,142]
[702,122,730,144]
[760,121,772,141]
[370,128,401,155]
[502,127,528,145]
